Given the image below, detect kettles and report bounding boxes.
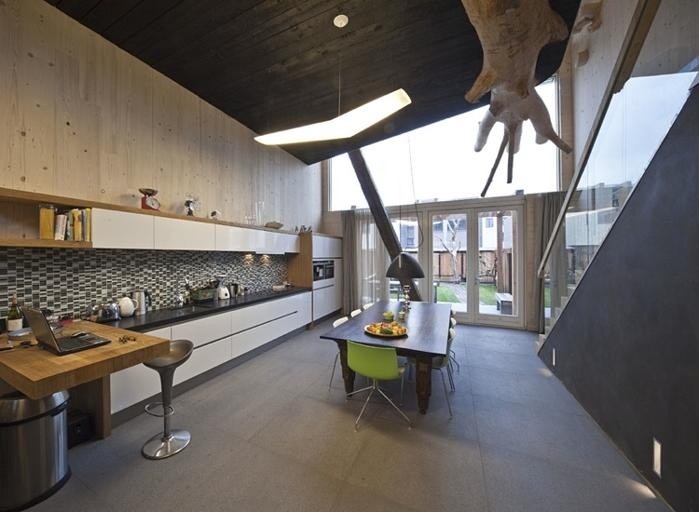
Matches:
[127,289,152,316]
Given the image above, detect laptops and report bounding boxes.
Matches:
[21,306,111,356]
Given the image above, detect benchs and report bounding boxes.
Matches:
[496,292,512,314]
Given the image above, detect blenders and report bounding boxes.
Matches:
[210,274,231,301]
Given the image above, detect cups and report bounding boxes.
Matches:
[398,312,405,321]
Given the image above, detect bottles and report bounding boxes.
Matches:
[6,292,24,332]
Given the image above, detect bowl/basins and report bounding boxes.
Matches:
[382,312,394,322]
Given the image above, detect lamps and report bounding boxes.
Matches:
[386,252,426,311]
[253,14,412,147]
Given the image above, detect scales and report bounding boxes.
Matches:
[139,188,160,211]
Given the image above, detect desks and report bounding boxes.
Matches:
[0,320,170,440]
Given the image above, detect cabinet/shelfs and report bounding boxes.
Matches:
[312,232,343,321]
[109,326,171,429]
[155,210,215,251]
[232,291,313,366]
[171,311,232,389]
[215,221,301,253]
[0,187,92,249]
[93,201,154,250]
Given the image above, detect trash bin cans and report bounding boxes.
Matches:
[0,389,72,512]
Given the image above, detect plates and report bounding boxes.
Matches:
[363,324,408,337]
[265,222,284,229]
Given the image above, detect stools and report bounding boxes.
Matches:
[141,340,194,459]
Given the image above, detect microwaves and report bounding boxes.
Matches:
[312,260,334,281]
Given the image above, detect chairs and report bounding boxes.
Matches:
[450,308,457,359]
[351,308,362,318]
[400,327,456,416]
[364,303,373,310]
[328,316,371,390]
[347,339,414,431]
[448,317,459,391]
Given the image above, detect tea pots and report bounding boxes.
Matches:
[102,301,121,321]
[116,296,139,317]
[229,282,239,298]
